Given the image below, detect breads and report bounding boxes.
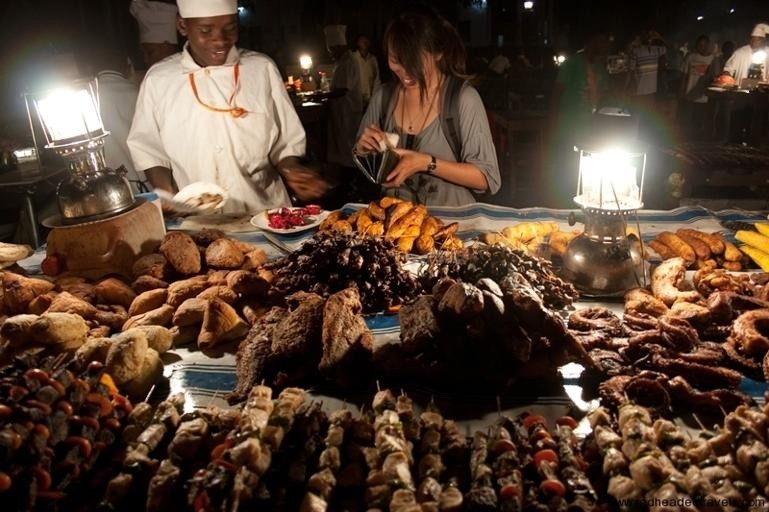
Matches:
[0,223,277,403]
[479,222,638,253]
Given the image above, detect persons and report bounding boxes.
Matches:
[352,34,379,115]
[305,25,366,204]
[126,0,331,214]
[91,0,180,195]
[352,13,501,207]
[546,23,769,151]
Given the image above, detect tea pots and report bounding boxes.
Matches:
[55,164,137,226]
[561,233,640,300]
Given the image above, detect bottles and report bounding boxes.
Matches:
[318,71,329,92]
[287,75,297,90]
[134,192,168,236]
[301,73,311,91]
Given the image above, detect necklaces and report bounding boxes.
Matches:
[405,89,436,132]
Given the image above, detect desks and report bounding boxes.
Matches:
[490,110,552,199]
[0,159,65,252]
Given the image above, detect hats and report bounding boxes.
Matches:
[176,0,238,19]
[750,23,769,38]
[129,0,178,44]
[323,24,347,49]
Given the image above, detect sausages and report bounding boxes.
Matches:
[646,226,747,273]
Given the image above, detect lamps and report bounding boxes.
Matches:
[24,78,137,226]
[561,146,646,303]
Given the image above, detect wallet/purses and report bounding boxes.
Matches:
[353,149,399,185]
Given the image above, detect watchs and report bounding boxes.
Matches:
[426,156,437,175]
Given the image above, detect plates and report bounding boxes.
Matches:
[41,197,149,229]
[251,205,334,234]
[171,180,227,214]
